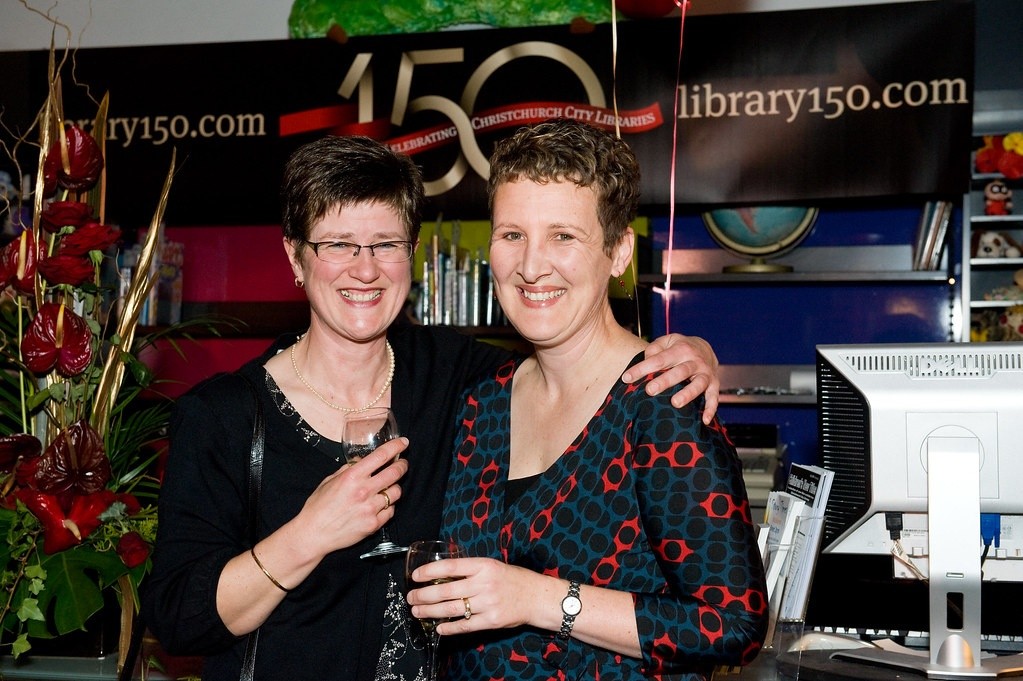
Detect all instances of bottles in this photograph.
[711,618,804,681]
[101,222,183,338]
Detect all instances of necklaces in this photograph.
[292,332,395,411]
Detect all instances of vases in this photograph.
[3,567,118,659]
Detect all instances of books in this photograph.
[420,234,509,326]
[913,200,953,271]
[755,462,835,622]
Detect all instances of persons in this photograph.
[406,116,769,681]
[142,134,719,681]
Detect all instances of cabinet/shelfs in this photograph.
[638,169,1023,405]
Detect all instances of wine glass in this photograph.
[342,407,410,558]
[405,540,463,681]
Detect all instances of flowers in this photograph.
[0,0,249,681]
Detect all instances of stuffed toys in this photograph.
[970,131,1023,343]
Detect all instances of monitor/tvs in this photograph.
[815,340,1023,681]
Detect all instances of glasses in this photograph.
[303,237,416,263]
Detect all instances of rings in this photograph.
[379,491,390,509]
[463,598,471,620]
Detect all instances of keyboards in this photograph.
[813,626,1023,652]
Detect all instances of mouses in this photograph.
[785,631,876,653]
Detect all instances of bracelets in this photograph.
[251,548,291,593]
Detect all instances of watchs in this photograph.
[557,580,582,639]
